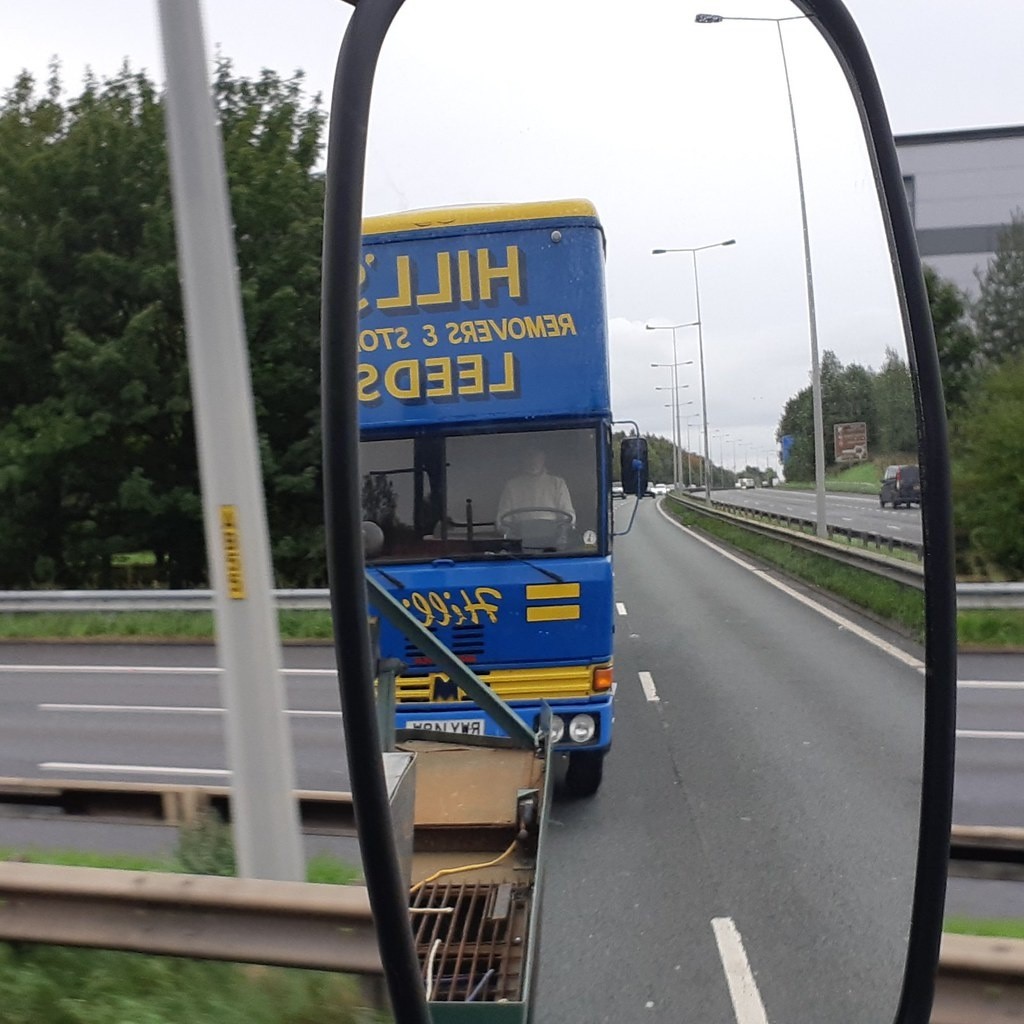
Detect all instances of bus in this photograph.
[346,195,647,805]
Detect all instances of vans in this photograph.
[878,464,923,510]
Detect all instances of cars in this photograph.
[666,484,675,493]
[655,484,668,495]
[746,479,755,488]
[609,480,626,500]
[643,480,656,499]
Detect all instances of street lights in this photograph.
[648,322,701,498]
[653,240,735,500]
[664,401,775,489]
[655,384,689,494]
[693,13,828,537]
[651,361,693,497]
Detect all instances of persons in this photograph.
[496,437,575,536]
